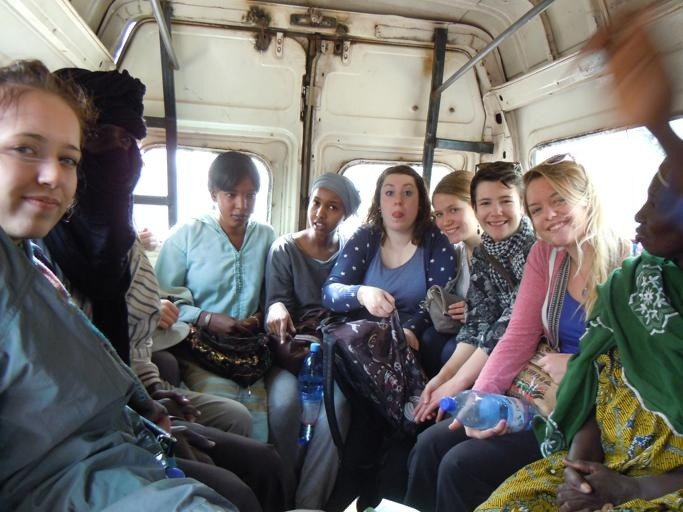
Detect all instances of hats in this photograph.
[149,313,191,353]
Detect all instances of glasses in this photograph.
[542,152,576,168]
[474,160,520,173]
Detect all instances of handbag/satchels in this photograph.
[418,284,465,334]
[271,305,330,372]
[317,309,427,458]
[507,343,573,417]
[183,330,270,389]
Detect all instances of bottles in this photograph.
[297,343,324,445]
[439,389,534,434]
[130,415,168,472]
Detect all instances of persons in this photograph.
[154,149,638,511]
[479,10,683,511]
[1,60,259,510]
